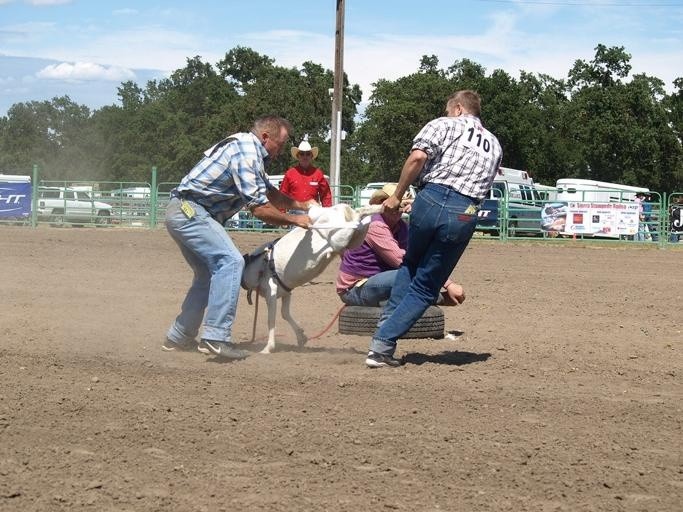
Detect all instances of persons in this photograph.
[632,192,682,243]
[364,90,504,366]
[163,115,319,360]
[277,139,333,229]
[335,182,466,309]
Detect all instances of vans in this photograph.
[0,172,30,226]
[471,166,547,240]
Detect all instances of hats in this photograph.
[383,183,414,208]
[291,141,319,161]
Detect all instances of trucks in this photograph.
[553,180,653,242]
[66,171,330,229]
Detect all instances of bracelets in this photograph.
[443,282,454,290]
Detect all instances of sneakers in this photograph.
[365,350,407,368]
[439,290,461,306]
[196,338,249,359]
[161,336,200,352]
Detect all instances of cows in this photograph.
[239,199,415,353]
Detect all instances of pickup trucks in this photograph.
[30,186,113,229]
[356,181,415,217]
